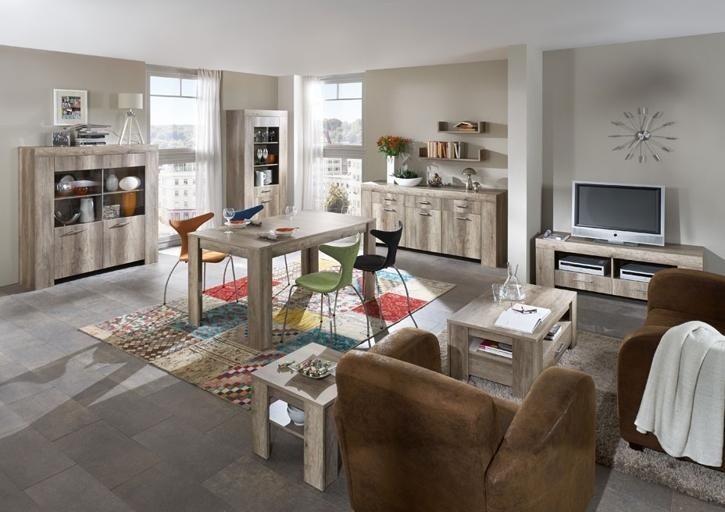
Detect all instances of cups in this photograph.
[492,283,504,305]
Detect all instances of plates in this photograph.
[224,221,251,230]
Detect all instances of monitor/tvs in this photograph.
[570,180,666,249]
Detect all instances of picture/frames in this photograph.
[53,88,88,127]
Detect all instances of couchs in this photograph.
[332,328,598,512]
[617,268,724,473]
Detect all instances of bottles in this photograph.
[500,262,525,300]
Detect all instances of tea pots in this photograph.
[255,170,266,187]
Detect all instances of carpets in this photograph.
[77,256,457,440]
[435,324,725,504]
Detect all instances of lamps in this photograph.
[117,93,145,145]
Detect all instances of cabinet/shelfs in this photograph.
[360,183,406,248]
[405,190,442,253]
[534,232,704,301]
[17,144,160,291]
[442,191,508,270]
[225,108,289,220]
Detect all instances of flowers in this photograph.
[375,135,414,158]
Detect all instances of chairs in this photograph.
[163,213,239,306]
[280,231,369,343]
[221,204,291,287]
[333,221,420,328]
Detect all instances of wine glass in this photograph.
[223,208,235,234]
[262,146,268,164]
[285,206,298,228]
[256,145,263,164]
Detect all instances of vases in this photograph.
[386,155,396,184]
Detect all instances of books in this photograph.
[477,339,513,359]
[427,141,466,159]
[544,324,562,341]
[494,303,551,334]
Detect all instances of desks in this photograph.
[186,210,377,351]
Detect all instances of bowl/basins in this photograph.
[274,227,295,238]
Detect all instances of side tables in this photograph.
[250,340,345,491]
